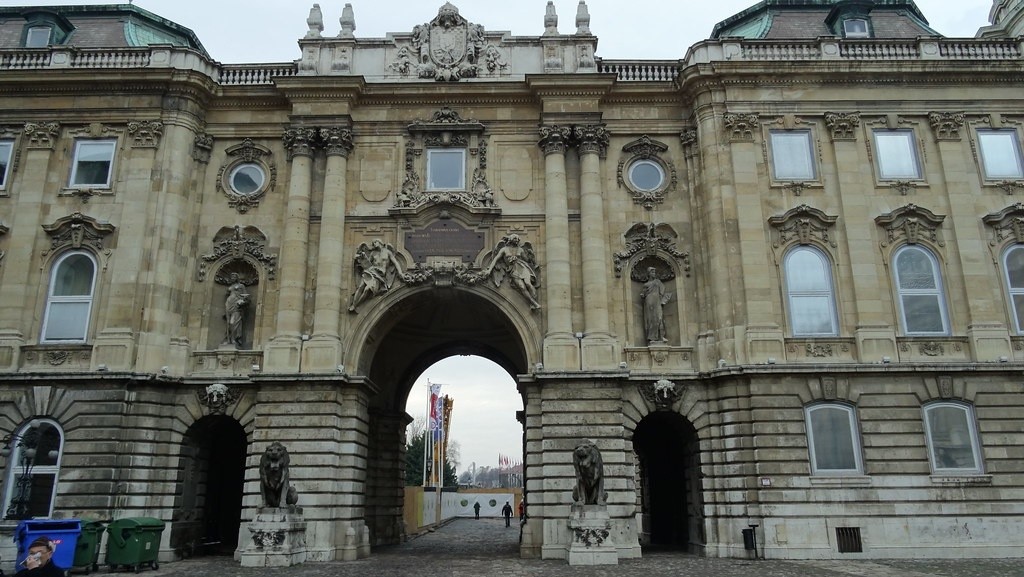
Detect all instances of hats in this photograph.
[27,536,57,553]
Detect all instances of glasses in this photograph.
[29,551,53,558]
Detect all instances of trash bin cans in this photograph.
[71,517,111,574]
[14,517,82,577]
[104,516,165,575]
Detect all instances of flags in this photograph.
[429,384,444,441]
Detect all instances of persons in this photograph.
[501,500,513,528]
[347,238,406,311]
[639,267,668,342]
[473,501,481,521]
[518,499,524,522]
[13,536,65,577]
[225,272,248,343]
[484,234,542,310]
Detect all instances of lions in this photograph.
[259,440,300,509]
[572,437,610,508]
